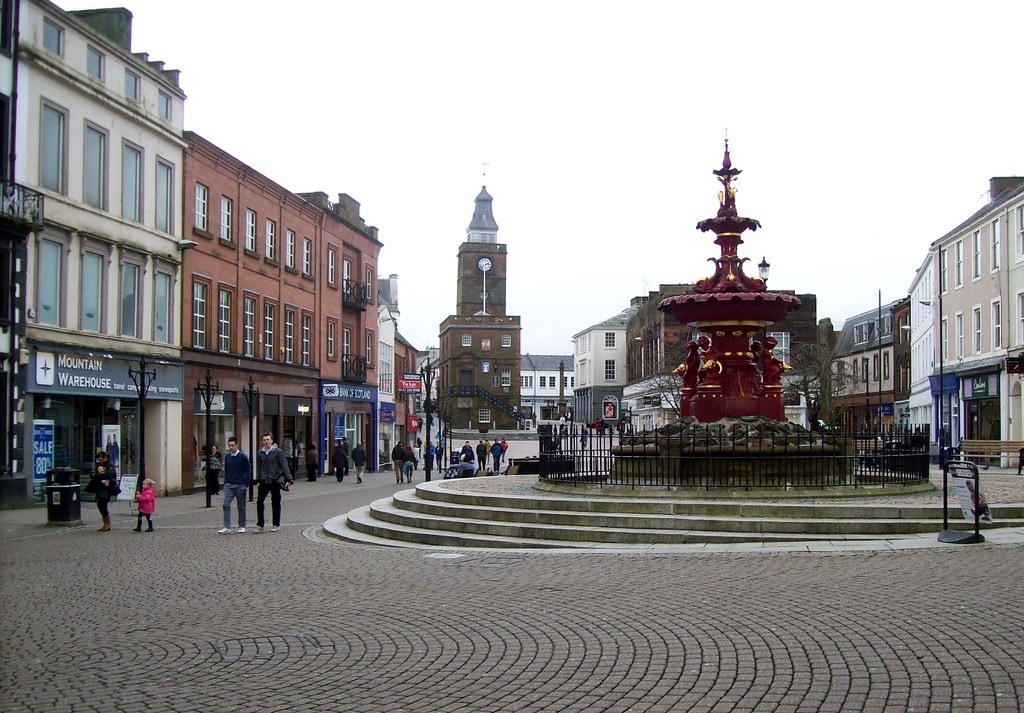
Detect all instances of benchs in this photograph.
[856,440,884,456]
[942,437,1023,474]
[500,458,576,475]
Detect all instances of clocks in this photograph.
[476,257,492,272]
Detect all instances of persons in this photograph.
[966,481,990,521]
[209,445,223,495]
[133,479,156,532]
[305,445,318,481]
[295,440,301,471]
[392,436,444,483]
[106,434,119,467]
[84,452,121,531]
[430,415,433,429]
[594,418,605,435]
[617,419,631,436]
[253,433,292,532]
[217,437,252,534]
[417,415,423,433]
[581,429,588,448]
[679,336,720,387]
[461,438,508,471]
[331,437,366,484]
[532,413,536,427]
[750,337,783,384]
[125,446,135,464]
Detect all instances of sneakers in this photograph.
[218,527,231,534]
[270,525,281,532]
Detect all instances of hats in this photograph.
[342,437,346,441]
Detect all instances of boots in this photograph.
[133,519,142,532]
[145,520,153,532]
[101,516,112,531]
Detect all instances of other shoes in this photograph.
[357,482,359,483]
[358,477,362,482]
[397,479,399,483]
[212,492,220,495]
[254,526,264,532]
[238,527,245,533]
[307,479,316,481]
[401,479,404,483]
[408,480,411,483]
[343,473,348,476]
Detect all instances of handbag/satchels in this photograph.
[276,474,289,491]
[403,464,408,475]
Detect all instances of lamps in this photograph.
[901,325,911,331]
[175,239,199,252]
[43,397,51,408]
[494,362,499,372]
[113,399,123,410]
[919,301,931,306]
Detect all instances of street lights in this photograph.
[920,299,945,469]
[193,368,220,508]
[862,351,870,437]
[421,357,435,481]
[241,373,260,501]
[127,355,157,517]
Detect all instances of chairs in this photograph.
[451,468,473,479]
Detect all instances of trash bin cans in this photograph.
[46,467,82,521]
